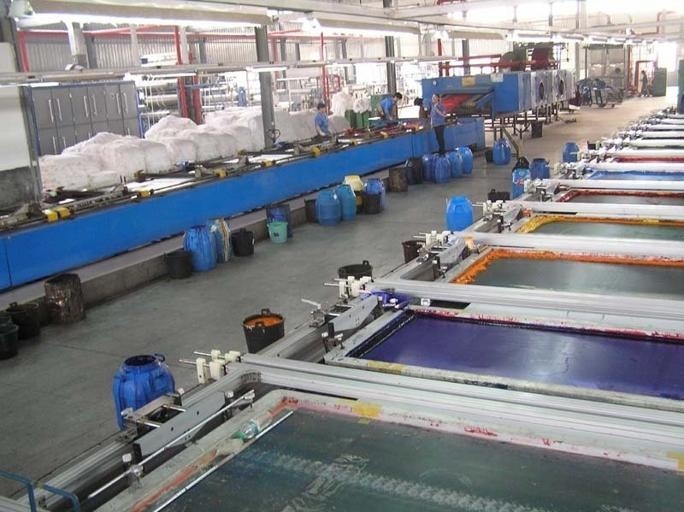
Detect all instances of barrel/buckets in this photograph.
[408,156,426,184]
[446,150,465,178]
[338,260,374,287]
[511,156,532,198]
[312,186,342,227]
[265,203,292,235]
[4,300,41,341]
[240,306,287,353]
[492,138,511,166]
[454,145,476,174]
[163,248,193,279]
[0,322,19,361]
[431,154,452,184]
[182,223,218,273]
[446,196,473,231]
[420,151,440,182]
[529,156,550,181]
[367,176,386,210]
[302,197,317,223]
[485,150,493,162]
[388,165,410,193]
[486,188,511,203]
[345,175,363,214]
[110,353,176,432]
[335,183,358,223]
[400,239,427,264]
[206,215,232,264]
[561,140,579,164]
[360,191,381,217]
[43,271,86,326]
[230,228,256,257]
[266,220,289,244]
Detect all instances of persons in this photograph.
[314,102,329,136]
[432,94,447,156]
[638,70,649,97]
[376,92,402,120]
